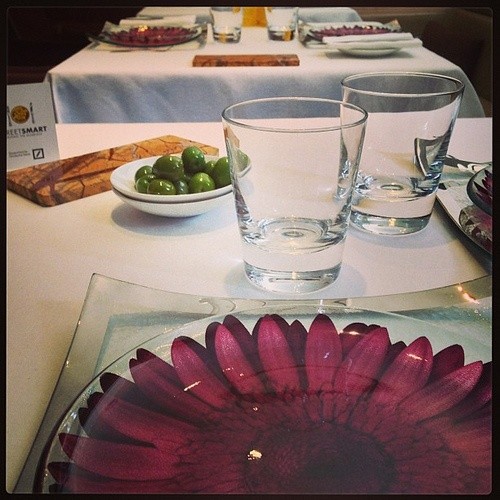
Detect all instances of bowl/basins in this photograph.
[109,154,252,219]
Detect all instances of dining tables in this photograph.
[7,100,492,494]
[137,5,363,25]
[44,19,489,124]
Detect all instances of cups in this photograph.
[338,72,466,237]
[264,7,298,41]
[220,98,370,295]
[209,7,243,44]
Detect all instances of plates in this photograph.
[39,304,492,495]
[413,138,493,256]
[337,47,401,59]
[12,271,494,496]
[302,20,394,42]
[88,26,202,48]
[466,164,493,216]
